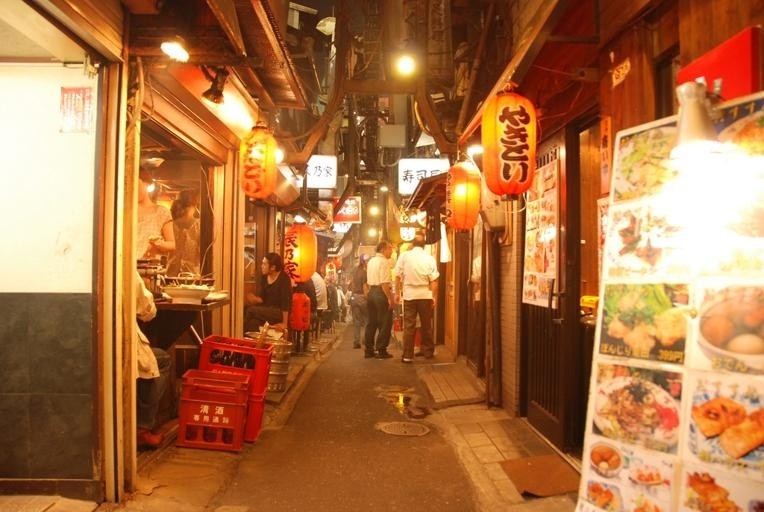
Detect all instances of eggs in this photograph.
[703,313,732,349]
[591,448,619,471]
[726,334,764,355]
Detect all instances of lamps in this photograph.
[444,83,536,232]
[399,222,417,241]
[200,64,231,105]
[239,99,275,199]
[667,78,728,163]
[159,24,193,64]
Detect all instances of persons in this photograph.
[293,234,441,362]
[471,256,485,381]
[136,270,164,447]
[243,253,290,334]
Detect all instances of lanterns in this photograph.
[240,125,278,204]
[445,156,482,232]
[480,90,539,199]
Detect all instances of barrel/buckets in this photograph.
[243,330,294,394]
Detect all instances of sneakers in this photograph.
[415,352,424,357]
[402,357,413,362]
[137,428,163,448]
[365,346,377,358]
[353,339,362,348]
[376,347,393,359]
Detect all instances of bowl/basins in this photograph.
[163,272,215,287]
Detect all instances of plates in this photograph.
[587,110,764,512]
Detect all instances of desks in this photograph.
[154,297,230,347]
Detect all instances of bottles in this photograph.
[212,349,254,368]
[137,269,155,296]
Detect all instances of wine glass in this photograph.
[159,287,210,306]
